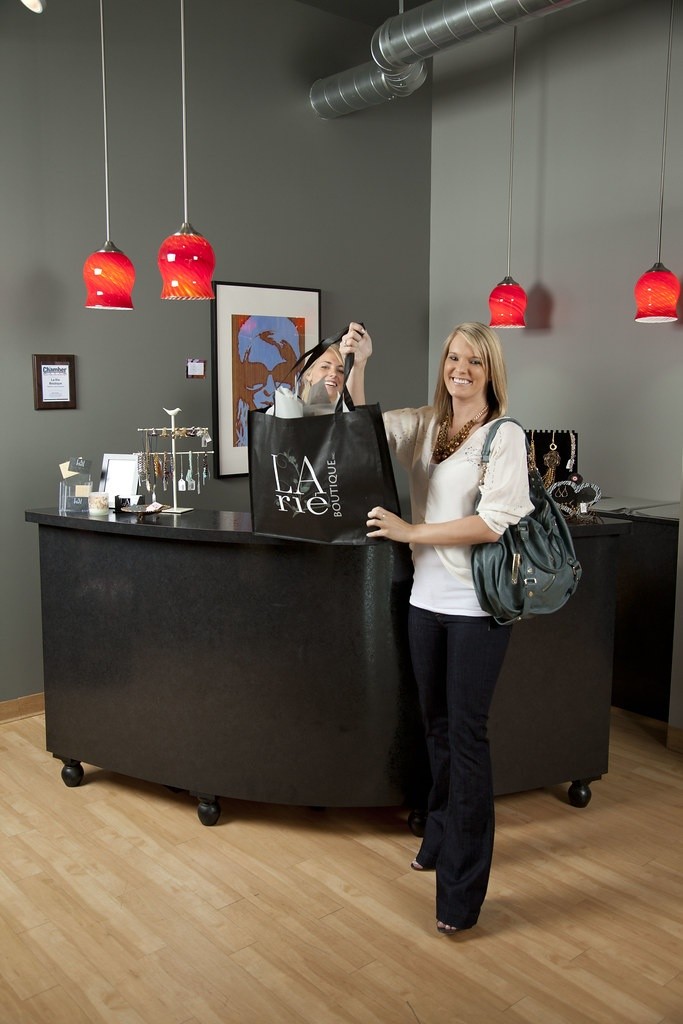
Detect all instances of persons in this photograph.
[339,321,535,933]
[299,339,344,408]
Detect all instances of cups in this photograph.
[88,492,109,516]
[75,484,93,498]
[114,494,145,513]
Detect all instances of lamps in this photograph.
[158,0,217,301]
[489,24,529,330]
[83,0,135,310]
[632,0,682,325]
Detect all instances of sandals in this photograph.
[435,919,459,935]
[411,859,433,871]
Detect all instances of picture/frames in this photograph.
[31,354,77,411]
[209,279,324,479]
[99,453,141,509]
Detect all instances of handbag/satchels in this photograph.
[470,417,583,625]
[247,320,403,545]
